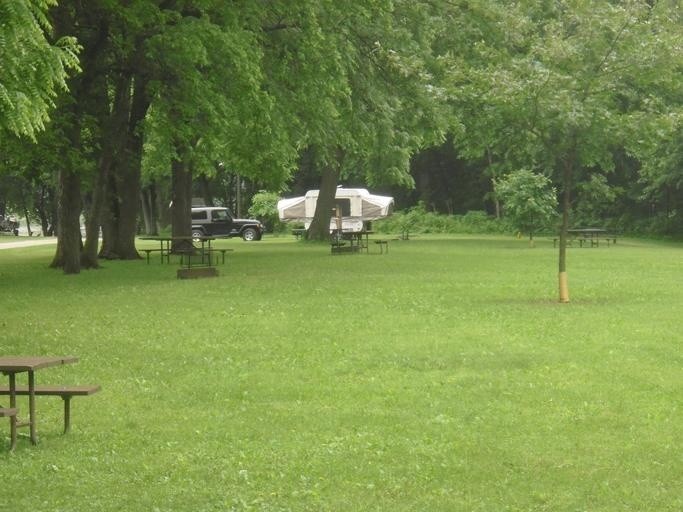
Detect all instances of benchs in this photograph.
[0,385,100,435]
[575,236,616,248]
[376,241,388,255]
[329,241,346,254]
[139,249,192,269]
[0,407,20,417]
[554,239,594,248]
[175,249,234,265]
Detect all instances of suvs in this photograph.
[190,207,264,243]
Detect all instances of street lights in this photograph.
[218,162,240,219]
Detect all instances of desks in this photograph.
[341,231,373,254]
[565,229,608,247]
[138,237,215,264]
[0,356,78,444]
